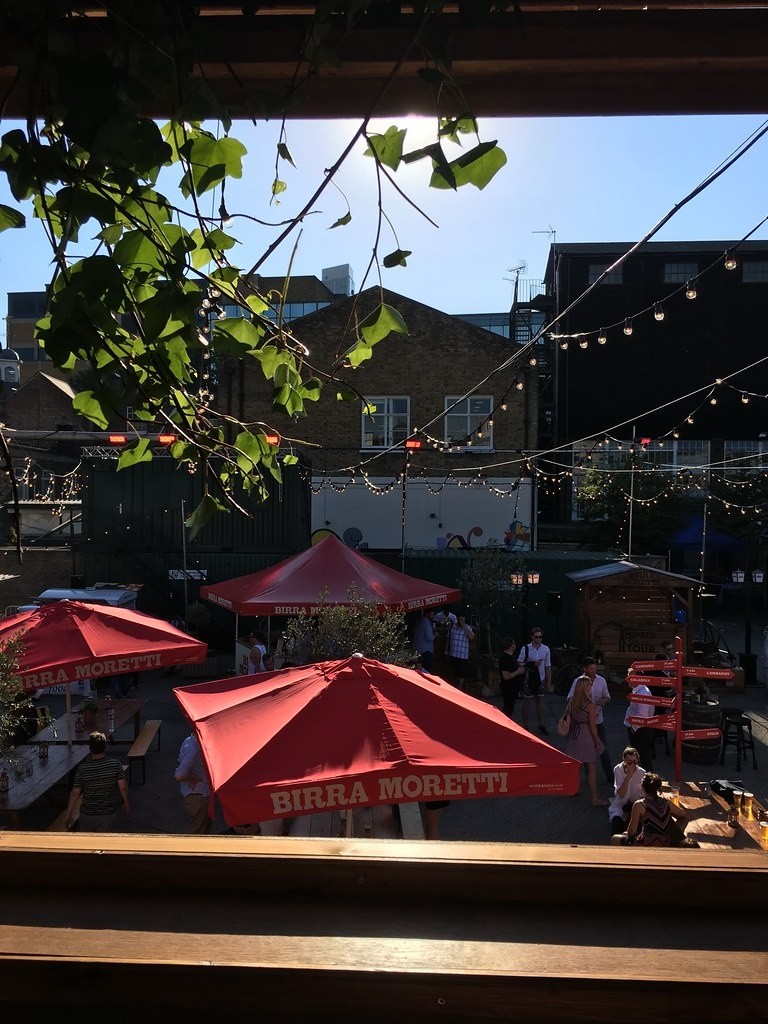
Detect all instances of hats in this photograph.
[251,631,265,641]
[89,730,108,752]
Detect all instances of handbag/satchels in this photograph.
[515,679,524,700]
[556,696,573,736]
[444,655,458,670]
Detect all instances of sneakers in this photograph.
[524,726,529,730]
[537,726,548,736]
[130,685,137,691]
[120,692,128,698]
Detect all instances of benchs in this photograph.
[45,764,129,832]
[126,720,163,787]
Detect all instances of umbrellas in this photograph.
[173,652,582,837]
[0,598,207,747]
[201,532,462,651]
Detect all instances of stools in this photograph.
[720,706,759,773]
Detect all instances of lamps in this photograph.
[265,434,280,446]
[159,434,176,444]
[109,434,126,445]
[404,439,422,448]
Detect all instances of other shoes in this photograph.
[606,776,614,782]
[608,832,612,839]
[591,799,609,807]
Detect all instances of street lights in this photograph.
[627,426,651,561]
[400,436,424,577]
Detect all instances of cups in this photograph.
[759,821,768,839]
[733,790,743,806]
[743,792,753,807]
[671,785,680,796]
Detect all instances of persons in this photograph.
[262,653,276,671]
[247,630,266,674]
[425,800,450,839]
[174,731,212,834]
[216,822,262,873]
[415,604,710,847]
[65,732,130,832]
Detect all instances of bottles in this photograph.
[75,696,94,732]
[594,744,603,755]
[108,707,115,731]
[104,692,112,720]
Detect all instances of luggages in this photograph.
[734,654,745,691]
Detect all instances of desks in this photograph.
[287,805,397,840]
[657,778,768,851]
[0,696,152,830]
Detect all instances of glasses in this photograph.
[664,647,674,652]
[249,635,255,638]
[225,668,236,673]
[623,759,639,764]
[458,618,465,621]
[533,635,543,639]
